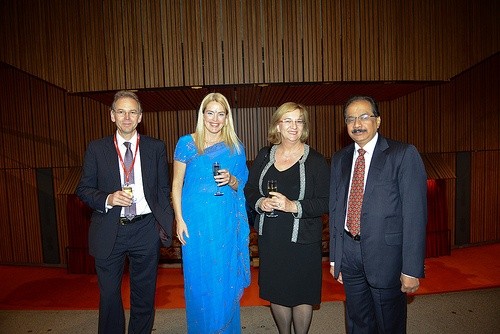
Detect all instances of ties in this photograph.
[123,142,137,221]
[346,149,366,238]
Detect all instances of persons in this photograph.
[242,102,330,334]
[171,93,251,334]
[77,92,175,334]
[329,95,428,334]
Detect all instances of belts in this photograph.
[344,229,361,241]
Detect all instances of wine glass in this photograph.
[211,161,224,196]
[122,187,136,217]
[266,178,279,218]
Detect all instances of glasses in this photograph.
[278,117,305,126]
[344,113,376,123]
[112,109,140,118]
